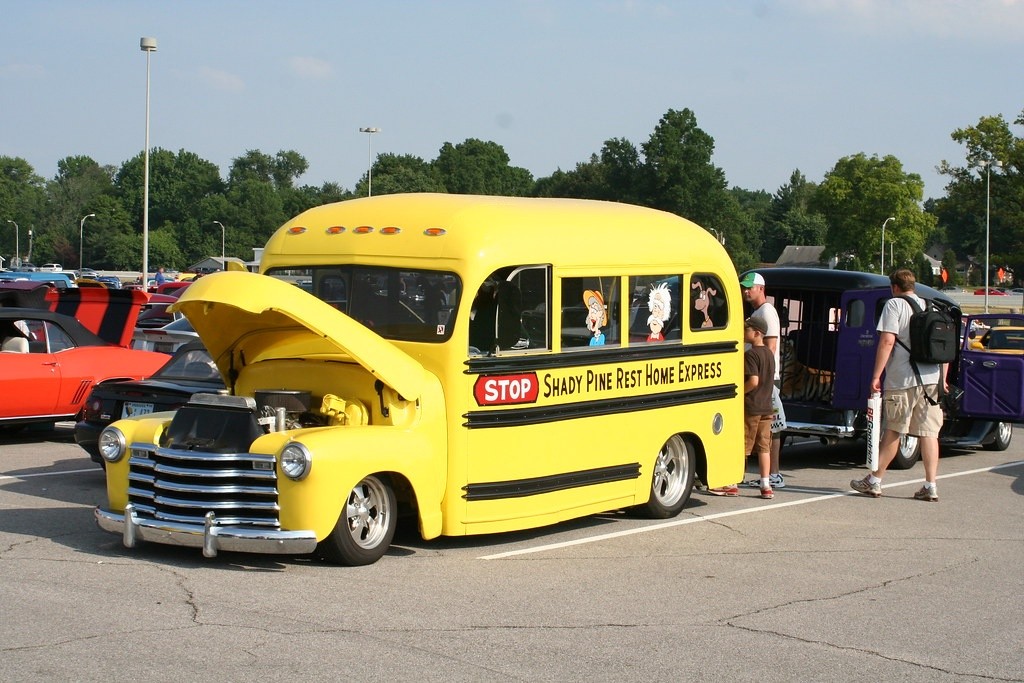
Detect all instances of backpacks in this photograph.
[894,295,955,364]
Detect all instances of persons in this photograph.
[155,267,165,286]
[708,316,774,498]
[850,270,949,501]
[740,272,788,487]
[193,268,205,282]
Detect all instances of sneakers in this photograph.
[914,485,938,500]
[749,474,785,488]
[760,485,774,498]
[708,484,738,497]
[850,478,882,498]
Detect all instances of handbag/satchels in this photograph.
[769,385,787,433]
[866,392,882,471]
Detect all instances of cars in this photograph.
[960,312,1024,355]
[0,263,228,472]
[936,285,1024,296]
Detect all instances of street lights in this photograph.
[79,214,95,278]
[139,37,158,289]
[881,217,895,277]
[7,220,18,267]
[978,154,1003,313]
[359,127,381,196]
[213,221,225,271]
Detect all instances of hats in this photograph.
[745,316,768,334]
[740,272,765,288]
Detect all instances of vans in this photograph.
[96,194,746,567]
[738,268,1024,470]
